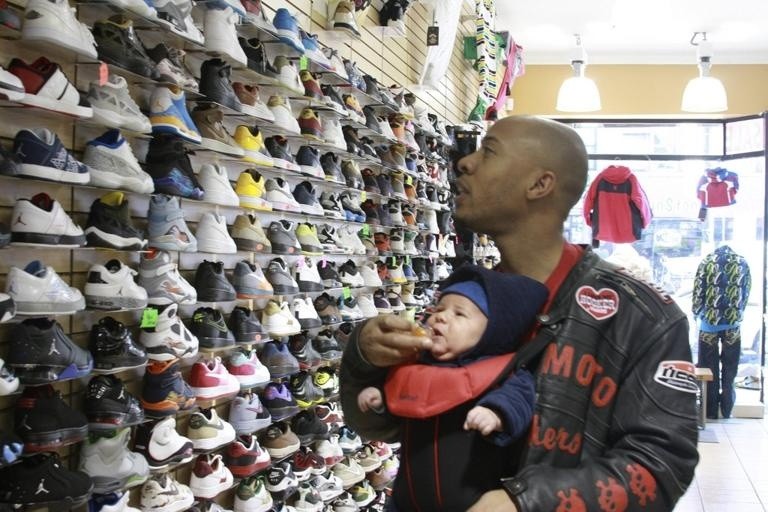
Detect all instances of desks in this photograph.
[695,366,714,425]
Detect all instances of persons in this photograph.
[694,245,752,420]
[338,115,698,512]
[357,266,550,510]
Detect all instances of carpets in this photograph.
[697,427,718,442]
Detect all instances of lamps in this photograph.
[556,33,602,113]
[680,28,729,111]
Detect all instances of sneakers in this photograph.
[233,475,274,512]
[134,248,199,308]
[193,210,238,255]
[376,126,467,228]
[272,5,306,59]
[289,295,323,330]
[9,191,89,252]
[229,392,274,437]
[341,94,368,125]
[355,292,380,319]
[197,162,241,209]
[90,11,161,82]
[299,27,332,72]
[1,1,22,43]
[189,356,242,403]
[187,453,235,501]
[317,224,355,256]
[263,256,300,296]
[193,260,238,304]
[336,427,364,454]
[359,168,381,196]
[385,286,407,312]
[241,0,280,36]
[1,357,19,399]
[311,366,341,396]
[75,425,152,496]
[136,301,200,363]
[286,445,328,482]
[296,222,326,257]
[290,255,326,293]
[233,169,273,213]
[318,150,349,187]
[87,488,144,512]
[229,212,272,255]
[337,191,368,223]
[320,117,348,152]
[364,466,392,491]
[81,126,156,195]
[140,358,197,420]
[348,479,377,507]
[335,226,368,256]
[343,57,367,93]
[143,132,205,202]
[146,80,205,145]
[207,0,247,18]
[385,255,408,285]
[232,121,274,169]
[237,33,281,82]
[272,500,296,512]
[331,455,367,489]
[358,198,381,227]
[266,218,303,256]
[292,481,325,512]
[312,433,344,466]
[333,0,362,41]
[298,69,326,105]
[403,229,500,284]
[319,82,350,119]
[374,231,394,257]
[150,41,201,94]
[0,56,94,122]
[12,383,92,447]
[8,318,95,386]
[200,502,234,512]
[357,260,383,288]
[299,109,326,144]
[289,370,326,412]
[319,189,348,222]
[0,427,25,465]
[184,405,238,456]
[380,0,412,35]
[2,290,15,326]
[84,372,147,431]
[311,328,344,361]
[271,55,306,98]
[259,421,302,461]
[373,289,393,313]
[341,124,366,159]
[366,75,438,130]
[337,158,366,192]
[226,348,272,386]
[199,57,242,113]
[232,259,275,301]
[82,190,149,252]
[6,126,90,186]
[354,445,383,474]
[85,315,149,376]
[336,294,363,323]
[289,409,331,446]
[266,94,301,137]
[1,450,94,511]
[110,0,205,50]
[313,400,345,431]
[357,226,380,256]
[129,415,195,471]
[8,259,88,319]
[315,259,342,289]
[289,180,326,218]
[87,70,153,136]
[263,459,301,502]
[21,1,103,65]
[226,431,271,479]
[404,285,435,309]
[295,145,327,182]
[260,298,302,338]
[260,340,300,378]
[357,134,383,165]
[308,469,345,504]
[260,380,299,423]
[231,79,276,124]
[200,5,249,70]
[381,455,401,477]
[229,305,271,344]
[263,132,302,176]
[337,259,365,288]
[313,291,343,327]
[285,334,322,371]
[330,491,360,512]
[190,307,236,350]
[323,45,348,80]
[83,257,148,313]
[264,177,303,216]
[139,472,197,511]
[145,192,200,255]
[373,259,395,286]
[334,322,355,349]
[369,440,393,459]
[190,102,246,160]
[389,226,407,256]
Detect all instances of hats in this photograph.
[433,280,489,317]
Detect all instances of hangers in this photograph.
[611,156,626,172]
[705,159,729,170]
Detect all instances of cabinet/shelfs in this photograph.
[0,0,503,511]
